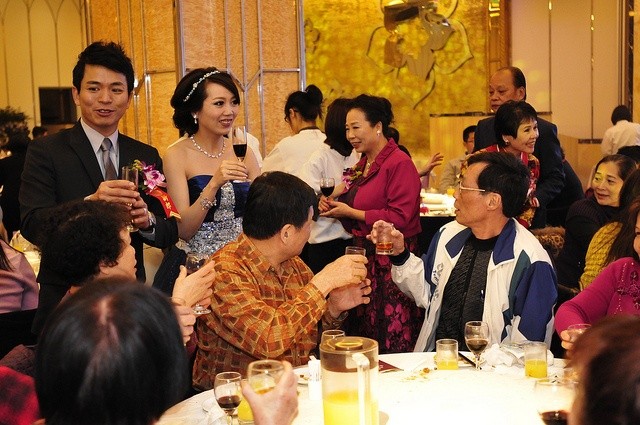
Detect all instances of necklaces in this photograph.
[367,159,374,168]
[190,134,225,159]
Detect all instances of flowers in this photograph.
[342,166,362,190]
[132,160,168,196]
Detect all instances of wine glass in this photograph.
[214,371,243,425]
[186,254,211,314]
[320,178,335,201]
[420,188,425,206]
[122,166,139,232]
[232,125,251,183]
[464,321,490,377]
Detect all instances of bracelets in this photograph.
[200,192,218,210]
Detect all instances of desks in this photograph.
[152,352,573,425]
[419,192,457,256]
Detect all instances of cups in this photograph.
[524,341,547,380]
[345,246,366,284]
[376,223,394,255]
[447,186,455,196]
[436,339,459,372]
[247,359,284,397]
[238,379,254,425]
[568,324,592,338]
[321,330,345,344]
[534,378,574,425]
[170,297,187,307]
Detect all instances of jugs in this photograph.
[319,335,380,425]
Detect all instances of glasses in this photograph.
[284,110,299,122]
[459,181,486,194]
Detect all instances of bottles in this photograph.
[12,239,23,253]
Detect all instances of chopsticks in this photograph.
[458,352,482,370]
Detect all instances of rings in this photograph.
[230,170,233,175]
[142,207,147,216]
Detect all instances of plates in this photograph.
[433,351,488,367]
[294,367,309,384]
[422,194,444,204]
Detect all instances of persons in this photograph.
[0,125,301,425]
[439,65,640,425]
[17,42,179,341]
[161,66,261,261]
[191,170,371,391]
[263,84,444,354]
[367,151,559,353]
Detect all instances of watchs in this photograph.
[140,211,156,231]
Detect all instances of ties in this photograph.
[101,138,118,181]
